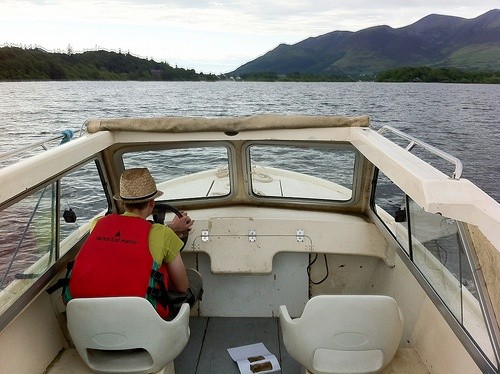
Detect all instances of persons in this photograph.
[89,166,203,320]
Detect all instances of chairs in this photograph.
[66,296,191,374]
[280,294,403,374]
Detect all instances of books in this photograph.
[227,343,280,374]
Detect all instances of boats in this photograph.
[0,116,500,372]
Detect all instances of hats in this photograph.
[112,167,164,204]
